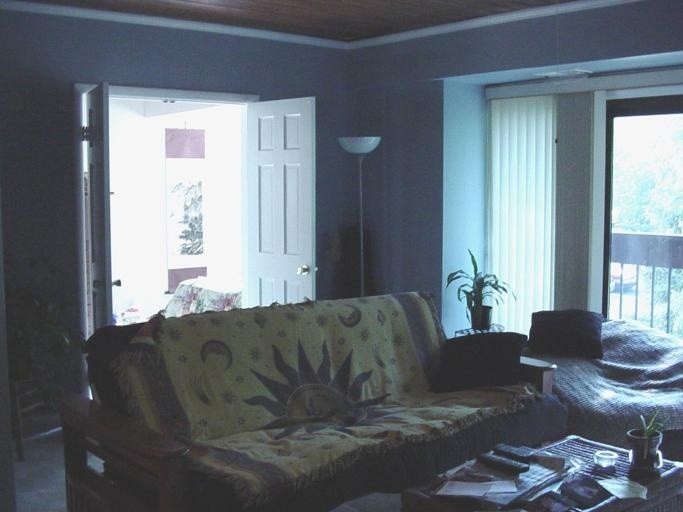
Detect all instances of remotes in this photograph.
[492,443,534,462]
[475,453,529,473]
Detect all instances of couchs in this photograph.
[63,291,570,512]
[527,312,683,461]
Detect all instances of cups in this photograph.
[594,450,619,467]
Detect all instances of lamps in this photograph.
[337,135,380,296]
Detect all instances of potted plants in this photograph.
[623,410,670,470]
[445,248,514,328]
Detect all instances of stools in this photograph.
[9,383,63,462]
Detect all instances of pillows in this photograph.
[528,310,605,359]
[428,332,527,392]
